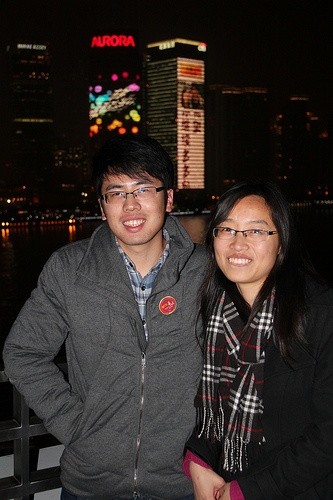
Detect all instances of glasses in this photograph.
[211,225,279,241]
[98,185,166,203]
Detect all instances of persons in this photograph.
[184,181,333,499]
[4,135,216,500]
